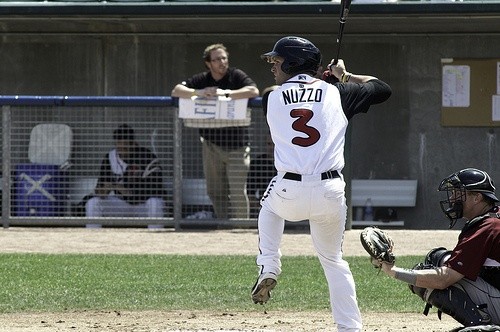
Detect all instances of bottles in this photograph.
[364,198,374,221]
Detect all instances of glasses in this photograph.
[209,56,230,63]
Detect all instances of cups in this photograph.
[356,207,363,221]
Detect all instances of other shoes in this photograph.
[457,321,500,332]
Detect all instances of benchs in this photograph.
[73,175,419,226]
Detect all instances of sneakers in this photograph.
[251,272,277,305]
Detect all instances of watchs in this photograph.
[224,89,231,98]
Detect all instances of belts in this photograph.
[283,170,340,181]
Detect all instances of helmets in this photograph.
[454,168,499,201]
[263,36,322,76]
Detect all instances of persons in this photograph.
[250,36,393,332]
[171,43,260,229]
[359,168,500,332]
[85,122,168,228]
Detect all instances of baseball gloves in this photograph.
[333,0,352,65]
[360,227,397,268]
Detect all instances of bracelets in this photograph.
[340,70,353,84]
